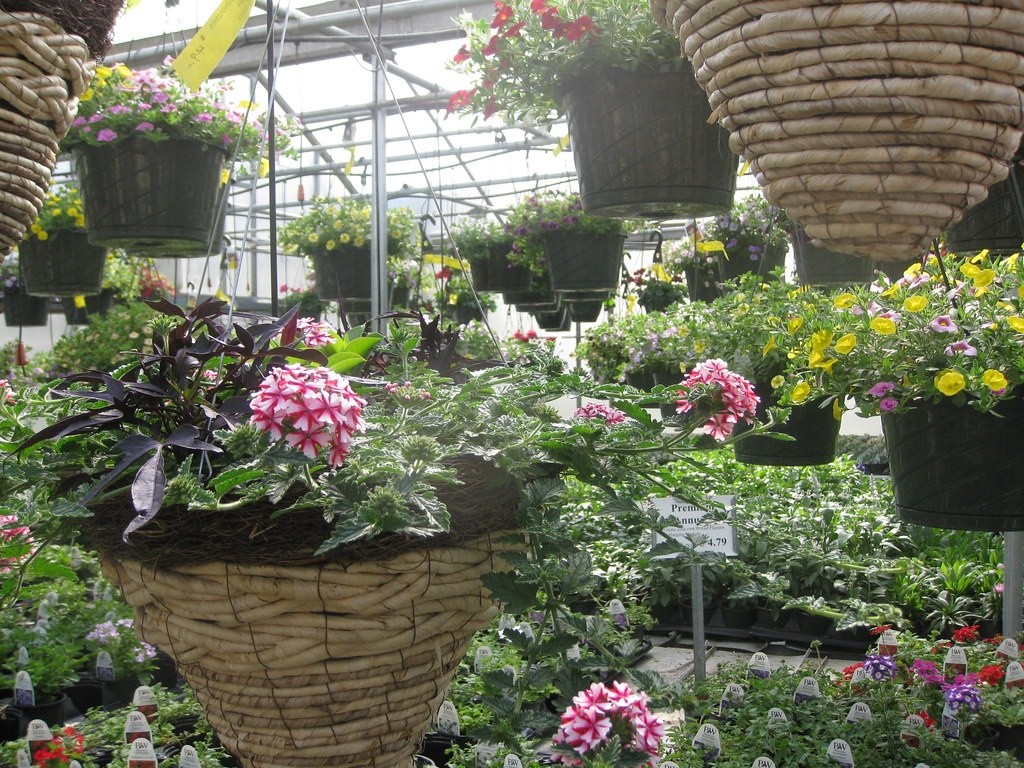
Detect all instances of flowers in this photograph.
[10,297,544,553]
[764,241,1024,417]
[504,194,587,276]
[454,216,514,263]
[384,253,434,289]
[0,251,21,293]
[23,180,88,242]
[841,623,1024,747]
[282,196,421,259]
[632,263,689,305]
[61,61,300,173]
[551,682,665,768]
[579,272,831,388]
[715,196,789,261]
[444,0,685,126]
[661,223,722,274]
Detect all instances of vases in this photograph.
[99,530,537,768]
[881,384,1024,531]
[312,250,339,300]
[567,301,603,322]
[626,370,660,408]
[65,132,231,252]
[388,282,410,310]
[561,290,609,302]
[730,242,785,283]
[733,386,845,466]
[60,288,112,326]
[646,299,672,314]
[337,244,371,301]
[686,263,733,302]
[967,721,1024,757]
[18,226,106,297]
[472,259,572,333]
[133,161,230,258]
[3,287,49,326]
[556,58,738,221]
[652,370,696,426]
[541,228,629,293]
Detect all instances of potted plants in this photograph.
[649,560,884,645]
